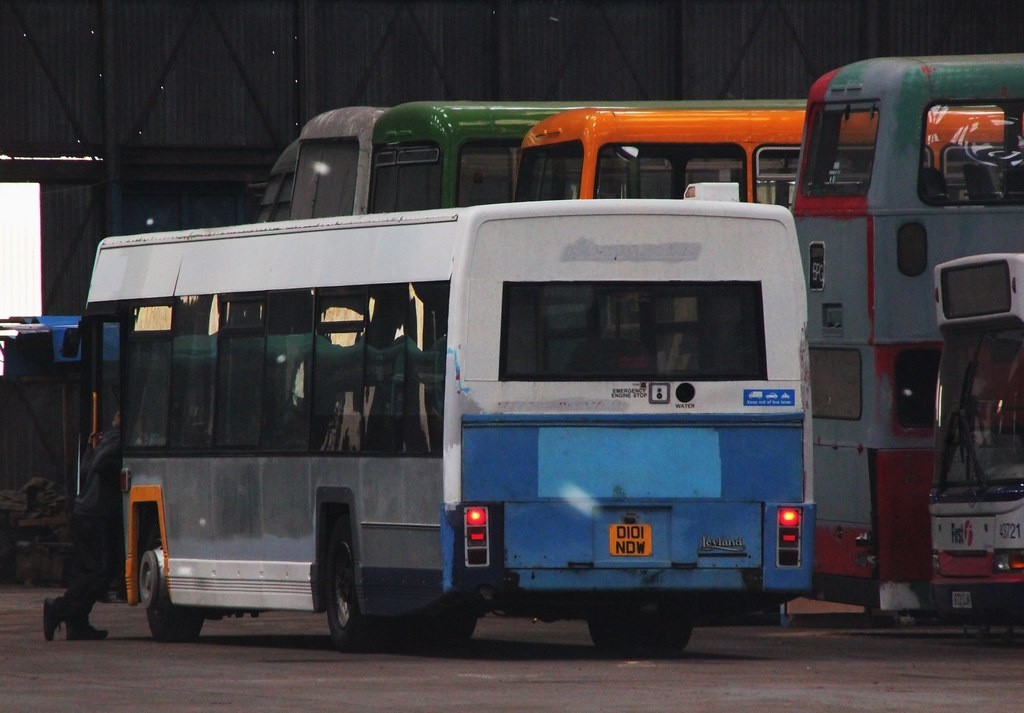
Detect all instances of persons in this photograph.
[43,410,120,641]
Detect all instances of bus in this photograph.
[58,177,818,661]
[502,105,1012,205]
[928,253,1023,638]
[287,96,388,217]
[365,96,807,218]
[788,49,1024,606]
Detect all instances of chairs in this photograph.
[963,162,997,200]
[921,169,947,202]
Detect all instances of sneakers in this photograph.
[65,614,108,641]
[43,597,64,640]
